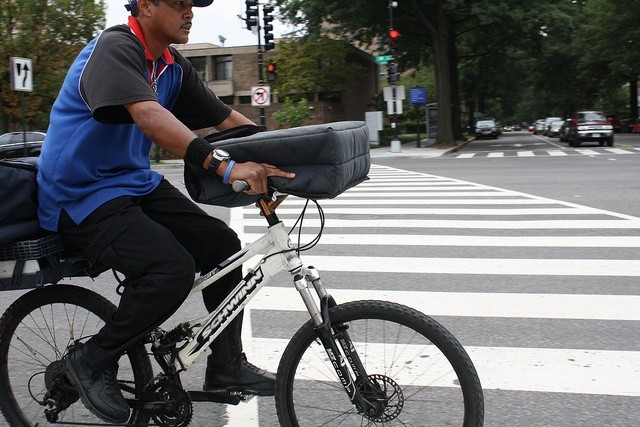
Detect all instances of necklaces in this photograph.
[151,60,158,93]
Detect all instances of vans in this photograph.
[484,116,501,135]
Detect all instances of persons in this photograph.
[35,1,294,423]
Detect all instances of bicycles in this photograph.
[1,177,486,426]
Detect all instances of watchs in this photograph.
[206,145,231,174]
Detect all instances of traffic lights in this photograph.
[387,28,400,56]
[245,0,258,31]
[264,4,274,51]
[266,61,275,83]
[389,63,400,82]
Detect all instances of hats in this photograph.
[124,0,214,11]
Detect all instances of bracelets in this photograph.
[223,159,236,184]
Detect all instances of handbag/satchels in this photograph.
[1,157,42,246]
[184,121,370,207]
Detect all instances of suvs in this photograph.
[606,113,624,134]
[544,117,562,136]
[569,110,615,147]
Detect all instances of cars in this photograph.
[0,132,45,156]
[549,120,565,138]
[504,125,512,132]
[514,124,522,131]
[474,120,498,139]
[560,118,573,142]
[528,122,535,131]
[536,119,544,134]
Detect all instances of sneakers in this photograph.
[203,353,277,396]
[64,342,130,423]
